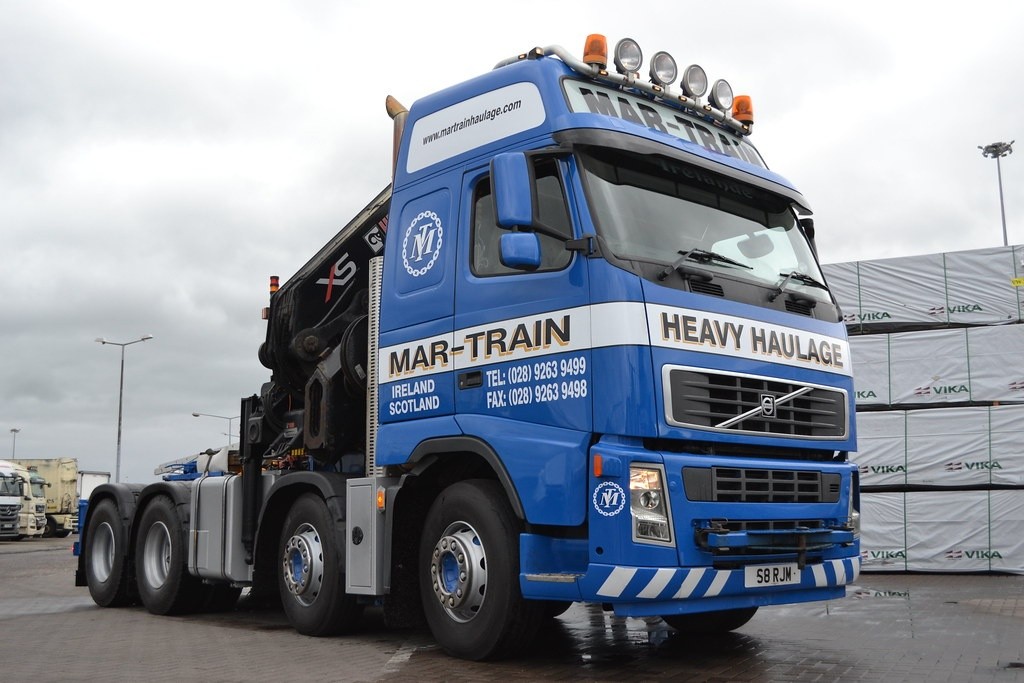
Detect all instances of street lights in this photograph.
[978,139,1015,247]
[94,333,154,484]
[192,413,240,446]
[10,427,21,459]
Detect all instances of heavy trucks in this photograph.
[0,457,77,542]
[72,32,864,662]
